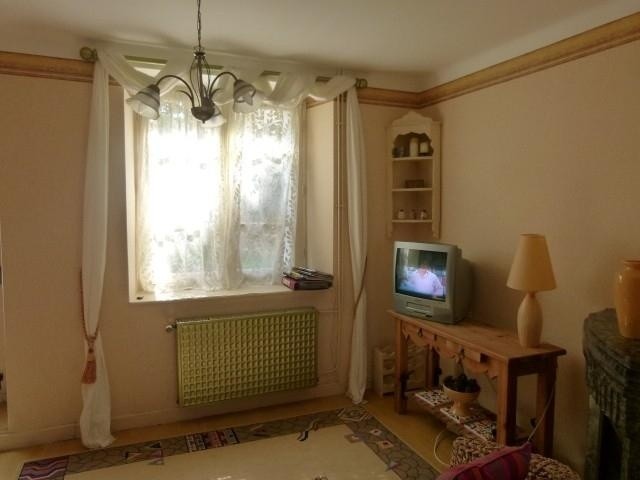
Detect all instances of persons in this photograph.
[406,259,442,297]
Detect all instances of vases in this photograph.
[614,259,640,339]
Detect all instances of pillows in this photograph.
[436,441,531,479]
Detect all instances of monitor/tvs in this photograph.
[391,240,475,324]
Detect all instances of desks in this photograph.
[582,308,639,480]
[384,309,567,459]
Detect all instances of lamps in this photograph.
[126,0,265,127]
[506,234,556,348]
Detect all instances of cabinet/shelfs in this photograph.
[385,109,442,239]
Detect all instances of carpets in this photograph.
[12,404,442,480]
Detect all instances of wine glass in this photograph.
[443,375,481,417]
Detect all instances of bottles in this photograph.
[409,137,419,157]
[399,209,428,220]
